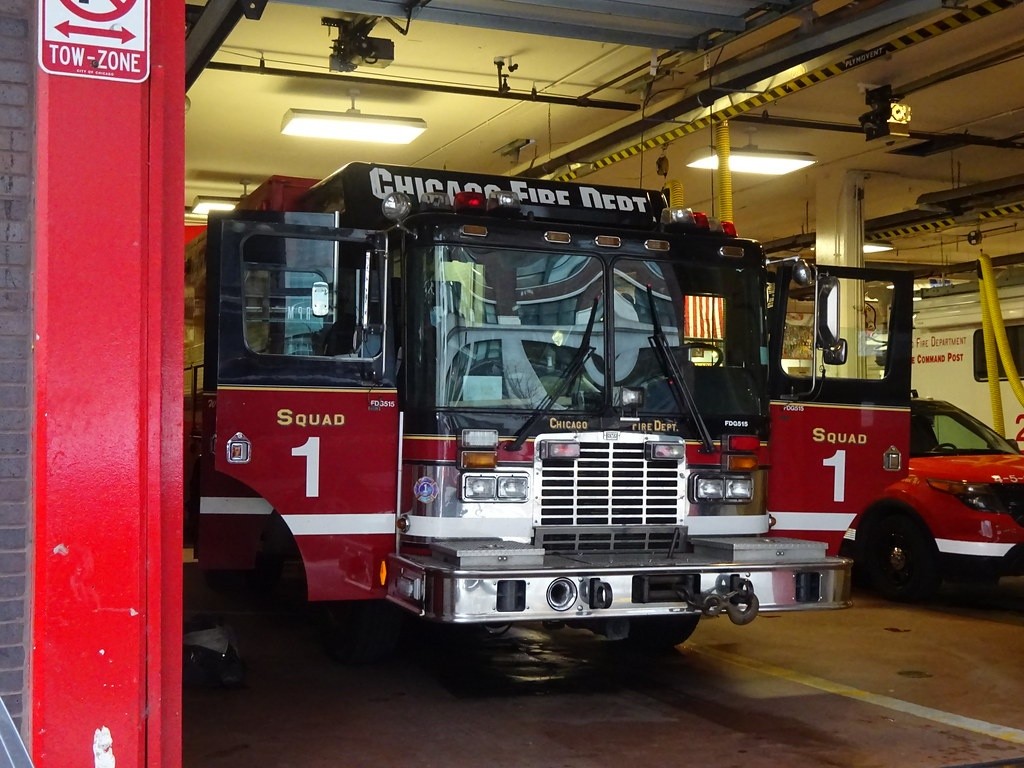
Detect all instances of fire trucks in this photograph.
[183,159,920,700]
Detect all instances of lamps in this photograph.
[278,83,428,145]
[191,178,249,219]
[811,184,896,256]
[686,125,819,178]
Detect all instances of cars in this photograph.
[841,382,1024,594]
